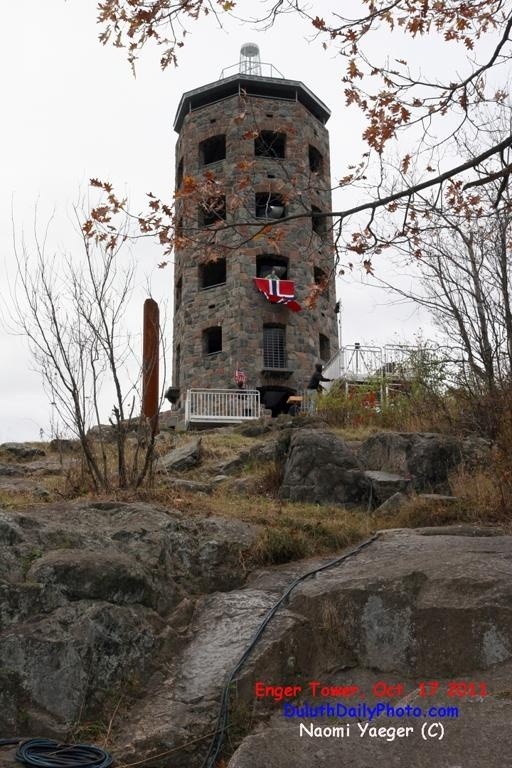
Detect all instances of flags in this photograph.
[254,279,303,312]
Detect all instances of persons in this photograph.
[307,363,335,415]
[264,270,280,280]
